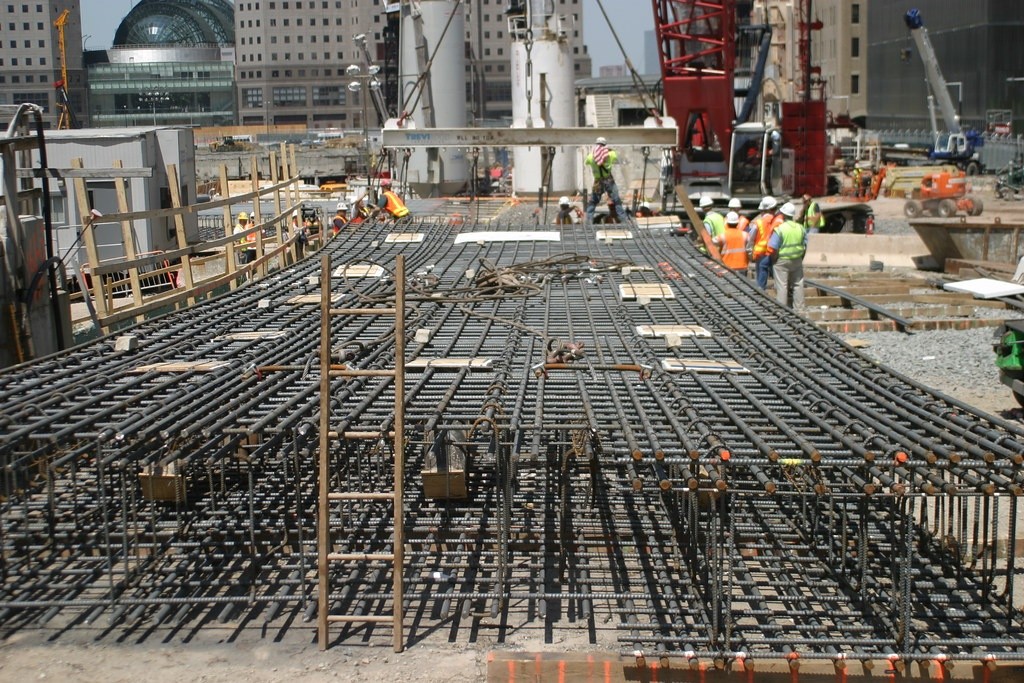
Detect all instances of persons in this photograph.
[853,162,862,198]
[699,193,824,311]
[291,210,306,257]
[334,203,348,237]
[233,212,264,276]
[557,196,580,224]
[585,137,628,225]
[378,180,409,224]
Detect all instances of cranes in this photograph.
[652,0,737,207]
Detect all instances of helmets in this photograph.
[728,198,741,207]
[699,196,713,206]
[337,203,348,211]
[762,196,776,210]
[802,194,811,202]
[758,202,763,210]
[727,212,739,224]
[237,212,248,219]
[250,212,254,217]
[292,210,298,218]
[559,196,569,204]
[780,203,794,216]
[596,137,607,145]
[380,180,392,187]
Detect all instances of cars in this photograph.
[299,140,322,146]
[284,138,302,144]
[319,184,347,196]
[260,184,322,199]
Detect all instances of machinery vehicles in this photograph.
[53,79,80,129]
[727,0,885,234]
[901,9,984,176]
[905,172,983,219]
[932,256,1024,410]
[300,203,324,234]
[351,34,391,129]
[209,134,245,152]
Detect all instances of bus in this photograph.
[233,135,252,142]
[317,133,343,142]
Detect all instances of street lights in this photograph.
[264,100,270,140]
[346,65,381,200]
[139,87,170,126]
